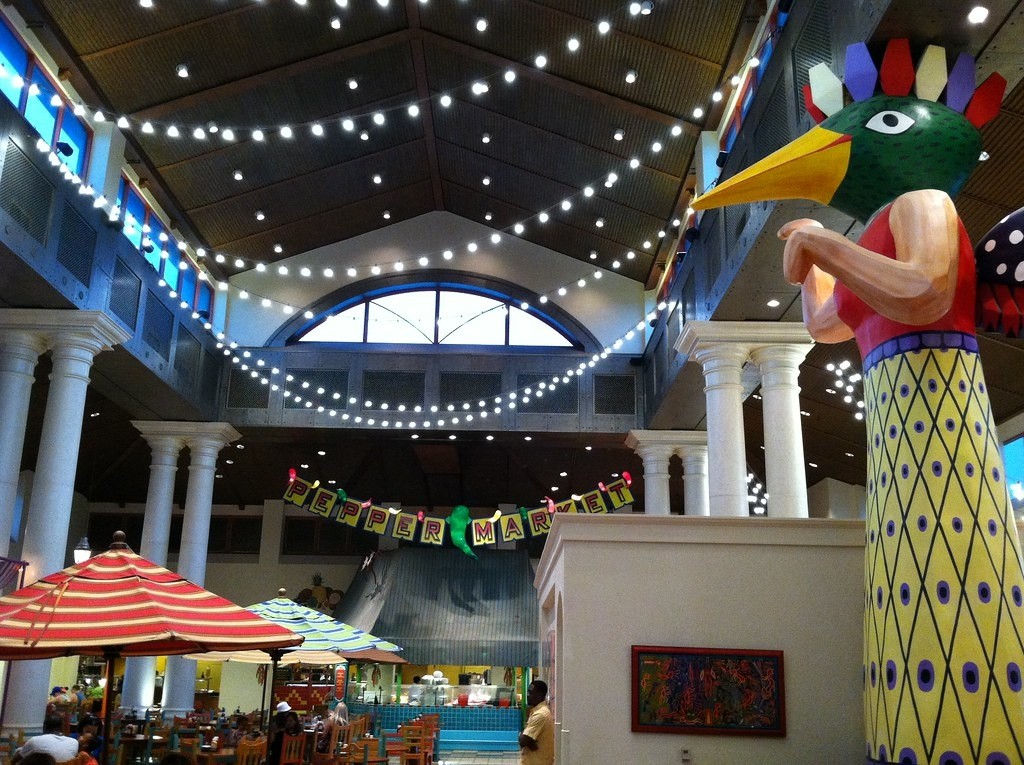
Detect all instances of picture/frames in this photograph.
[629,645,789,738]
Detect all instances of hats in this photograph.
[62,687,67,692]
[50,686,62,695]
[277,702,292,713]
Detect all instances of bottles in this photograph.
[211,738,218,749]
[8,736,17,758]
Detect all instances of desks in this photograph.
[408,727,440,737]
[120,717,157,731]
[351,755,387,764]
[178,719,252,727]
[381,745,409,764]
[155,724,211,739]
[302,730,326,762]
[406,734,435,762]
[170,746,250,764]
[109,732,166,764]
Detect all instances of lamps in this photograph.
[139,241,154,255]
[715,150,729,170]
[651,319,657,327]
[675,250,687,263]
[55,140,73,157]
[196,310,210,320]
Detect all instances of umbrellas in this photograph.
[183,651,348,732]
[332,649,410,704]
[0,531,305,765]
[245,588,404,765]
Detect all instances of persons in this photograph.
[12,685,104,765]
[317,702,348,754]
[409,676,423,703]
[268,701,298,765]
[518,680,554,765]
[229,716,249,745]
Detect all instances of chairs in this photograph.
[1,699,442,765]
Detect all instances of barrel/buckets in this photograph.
[458,694,469,708]
[499,697,510,709]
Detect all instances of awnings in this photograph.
[329,549,540,668]
